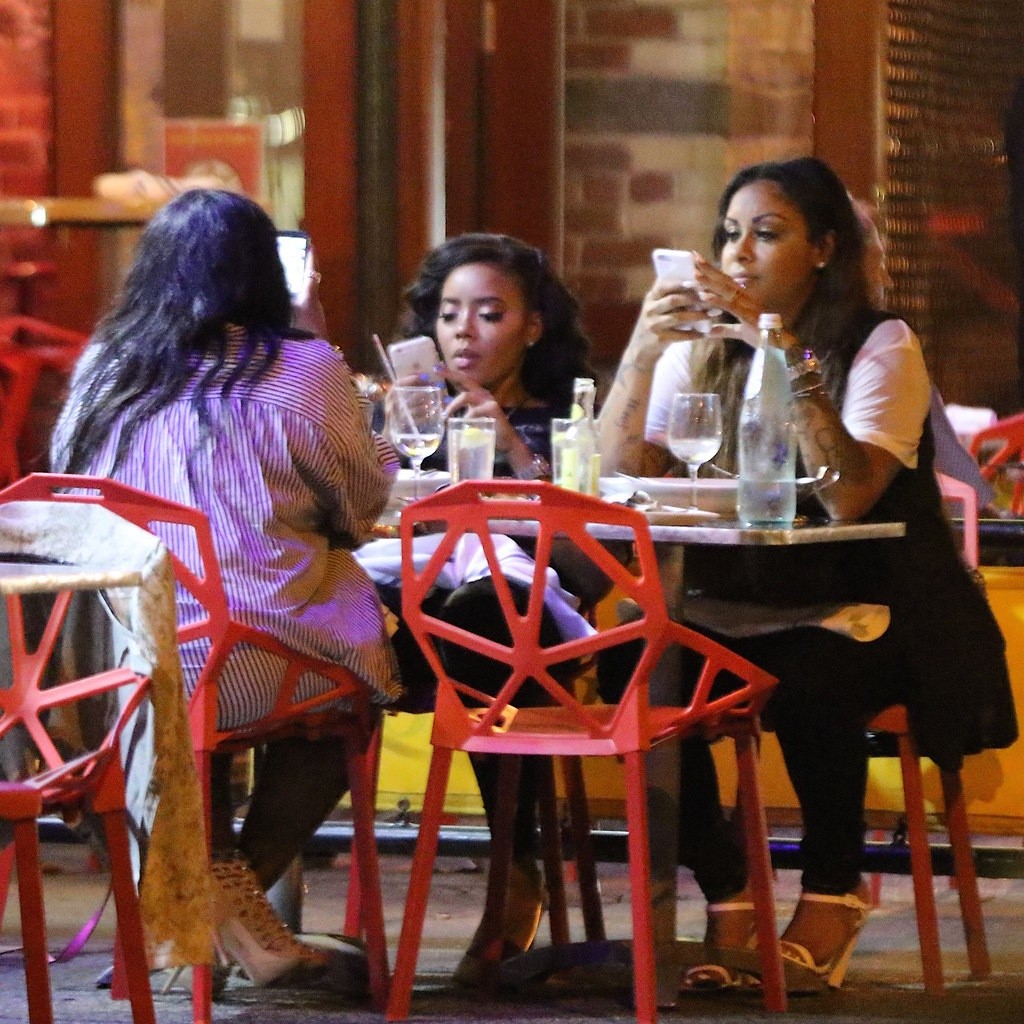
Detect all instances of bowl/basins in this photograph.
[600,477,734,510]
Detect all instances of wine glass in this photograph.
[388,387,445,499]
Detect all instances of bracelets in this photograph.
[783,345,834,404]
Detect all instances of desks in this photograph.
[0,561,143,594]
[380,519,911,1015]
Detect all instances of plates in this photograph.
[393,468,450,486]
[644,505,724,524]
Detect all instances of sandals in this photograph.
[446,868,545,999]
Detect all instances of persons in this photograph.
[594,156,1019,998]
[48,191,397,995]
[372,231,599,988]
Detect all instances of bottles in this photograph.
[563,378,598,497]
[737,312,796,522]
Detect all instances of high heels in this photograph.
[202,858,326,987]
[680,902,757,995]
[740,879,873,996]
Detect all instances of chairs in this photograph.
[0,473,386,1024]
[760,469,993,999]
[971,414,1024,517]
[386,478,788,1024]
[0,498,176,1024]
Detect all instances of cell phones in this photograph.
[277,231,312,307]
[389,336,454,429]
[650,248,715,333]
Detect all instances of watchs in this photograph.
[511,454,554,486]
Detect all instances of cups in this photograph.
[552,418,598,494]
[667,394,723,513]
[447,418,495,485]
